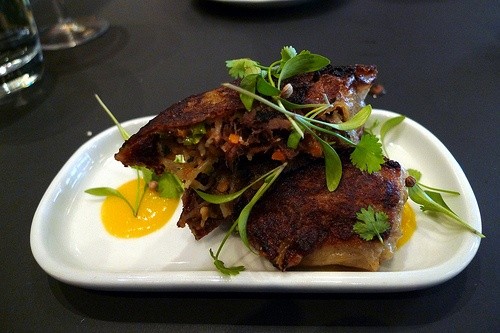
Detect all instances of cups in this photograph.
[0,0,44,98]
[30,0,110,51]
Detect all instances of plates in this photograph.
[30,109,482,293]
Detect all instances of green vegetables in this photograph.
[85,45,486,276]
